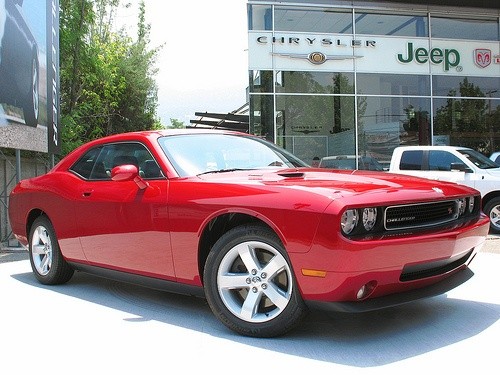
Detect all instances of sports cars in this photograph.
[7,127,491,337]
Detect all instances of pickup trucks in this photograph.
[317,154,385,174]
[349,145,500,235]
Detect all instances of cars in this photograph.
[0,0,40,126]
